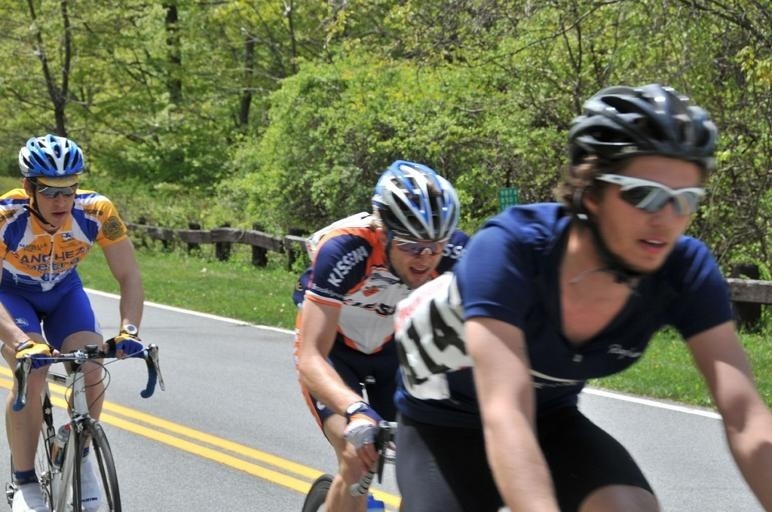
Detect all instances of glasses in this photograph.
[26,177,79,199]
[390,236,445,258]
[592,170,707,217]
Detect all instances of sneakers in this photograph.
[10,480,50,512]
[72,459,102,512]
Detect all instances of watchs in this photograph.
[341,402,377,421]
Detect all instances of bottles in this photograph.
[51,424,71,470]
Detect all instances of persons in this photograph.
[387,86,772,512]
[0,133,143,512]
[291,161,474,512]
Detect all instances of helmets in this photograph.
[19,135,85,178]
[372,158,460,244]
[565,82,719,173]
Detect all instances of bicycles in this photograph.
[5,340,165,512]
[300,416,403,512]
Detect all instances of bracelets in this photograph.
[117,324,138,336]
